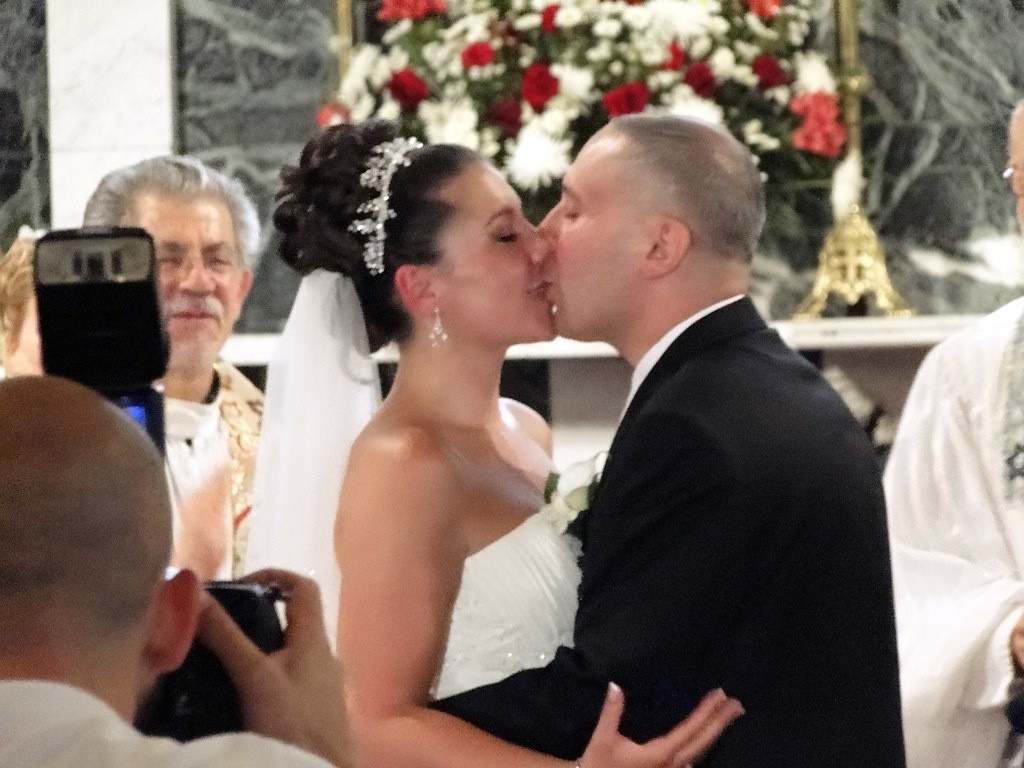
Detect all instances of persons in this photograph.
[273,114,908,768]
[882,97,1024,768]
[0,156,357,768]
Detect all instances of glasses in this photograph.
[1002,161,1024,197]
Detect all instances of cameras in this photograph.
[34,224,287,745]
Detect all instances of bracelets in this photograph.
[575,759,580,768]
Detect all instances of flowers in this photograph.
[552,449,610,533]
[315,0,854,255]
